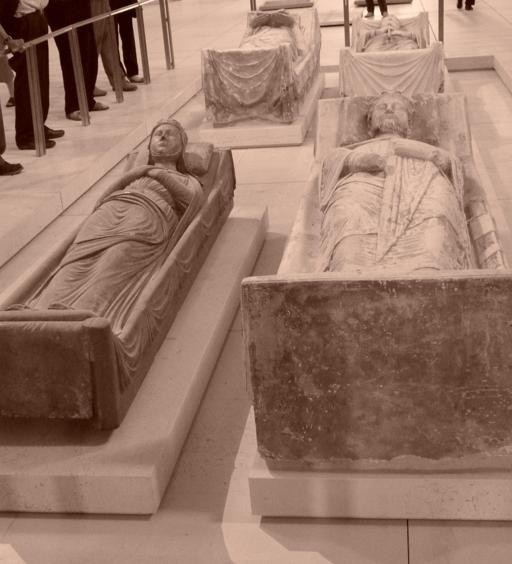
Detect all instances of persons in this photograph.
[356,13,420,53]
[316,89,473,278]
[238,7,298,66]
[1,0,148,178]
[363,0,389,16]
[455,0,475,10]
[4,115,204,323]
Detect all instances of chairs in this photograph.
[365,12,374,17]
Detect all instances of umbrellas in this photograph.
[0,75,144,176]
